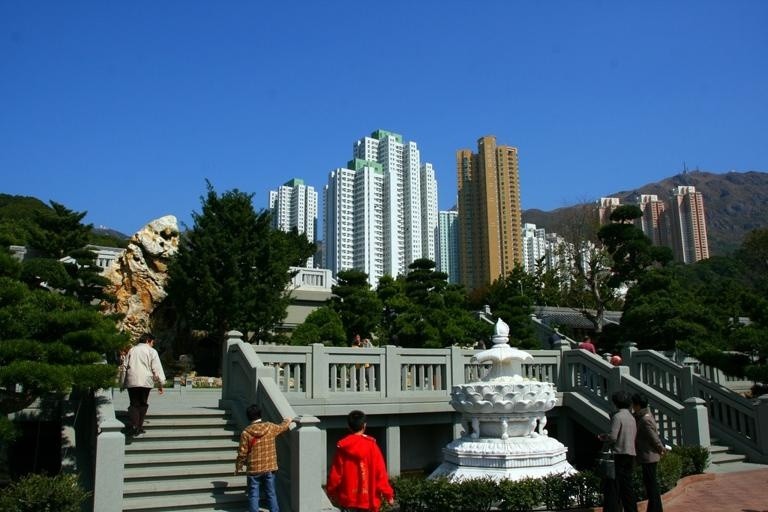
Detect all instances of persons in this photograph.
[327,409,395,511]
[577,336,596,388]
[628,392,667,512]
[118,329,167,438]
[609,352,623,366]
[233,403,294,512]
[596,389,642,511]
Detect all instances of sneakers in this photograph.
[128,422,145,437]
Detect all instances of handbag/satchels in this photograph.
[591,435,618,480]
